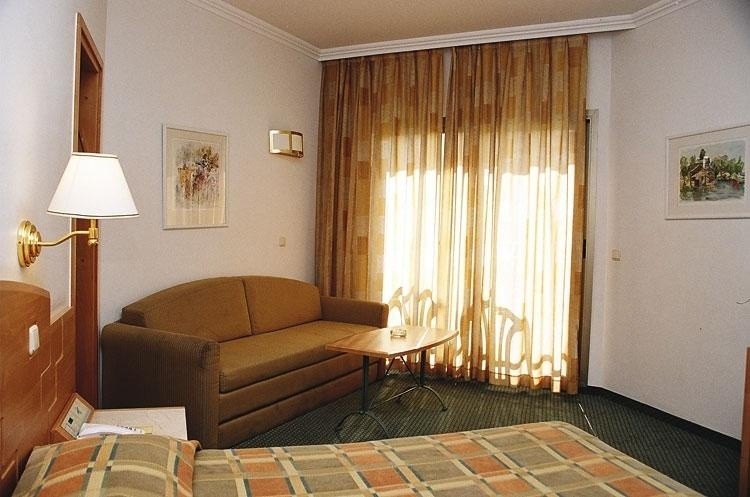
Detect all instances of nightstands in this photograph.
[52,393,188,440]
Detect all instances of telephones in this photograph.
[78,423,145,437]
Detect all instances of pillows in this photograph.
[7,434,201,497]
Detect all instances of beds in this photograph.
[1,281,711,496]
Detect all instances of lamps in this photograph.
[269,130,304,158]
[17,151,141,269]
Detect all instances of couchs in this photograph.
[100,275,389,448]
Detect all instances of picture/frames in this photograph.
[162,123,228,231]
[664,123,750,219]
